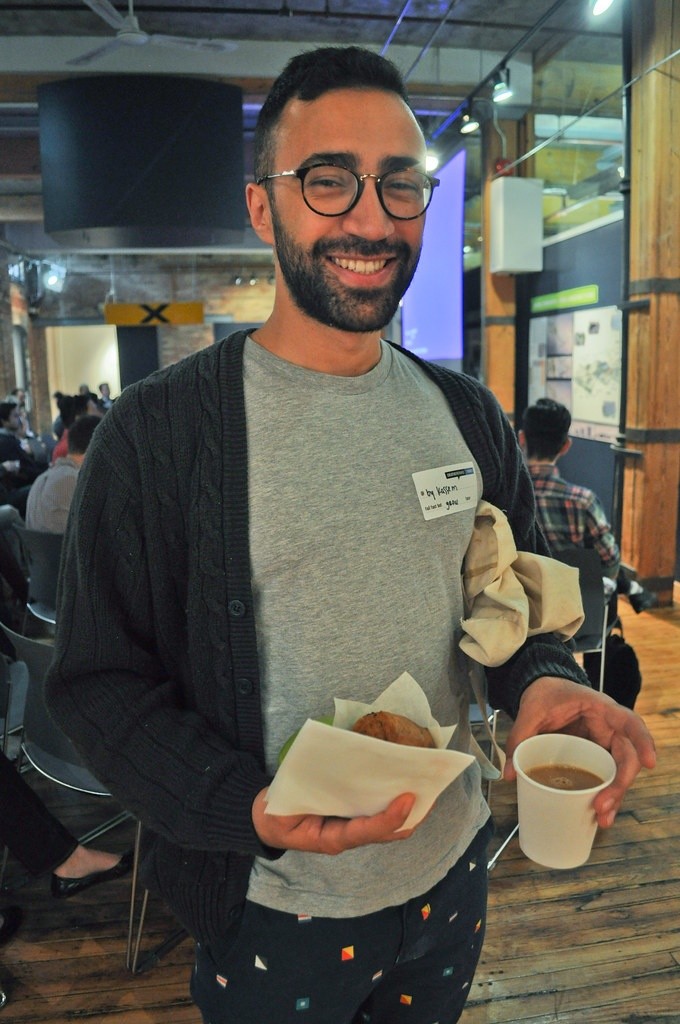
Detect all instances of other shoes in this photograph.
[610,616,624,639]
[628,585,659,614]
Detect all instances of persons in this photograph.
[47,47,657,1024]
[1,385,657,1023]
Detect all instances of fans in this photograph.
[66,0,238,70]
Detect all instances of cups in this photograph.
[513,732,617,868]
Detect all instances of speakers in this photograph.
[489,177,544,274]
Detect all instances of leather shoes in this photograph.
[51,847,134,898]
[0,904,23,945]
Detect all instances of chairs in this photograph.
[27,436,49,466]
[470,703,519,876]
[41,429,57,462]
[0,505,36,636]
[2,520,64,638]
[0,652,30,770]
[551,546,623,693]
[1,623,185,971]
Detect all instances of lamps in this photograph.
[460,108,479,135]
[234,266,275,286]
[38,259,67,293]
[7,258,42,302]
[492,62,514,103]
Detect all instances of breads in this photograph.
[351,711,435,748]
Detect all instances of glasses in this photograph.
[254,162,441,220]
